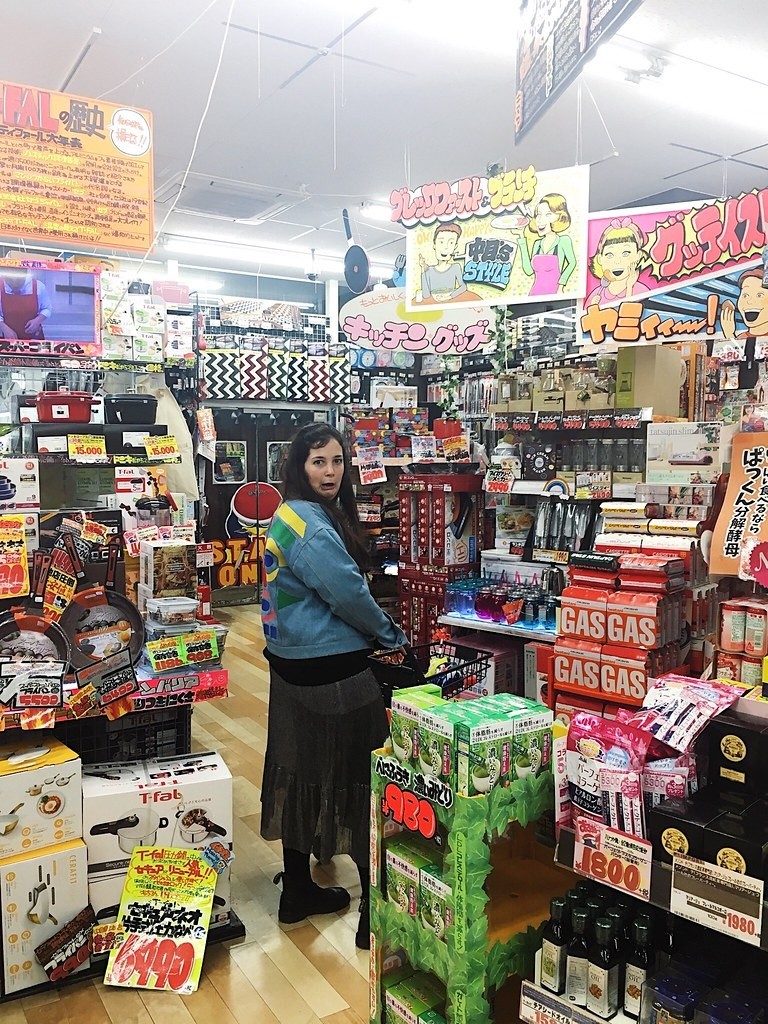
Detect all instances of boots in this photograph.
[355,897,370,950]
[273,864,351,924]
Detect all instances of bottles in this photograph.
[559,439,645,472]
[646,562,719,680]
[541,879,669,1022]
[443,577,562,631]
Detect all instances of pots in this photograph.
[174,808,227,843]
[90,807,168,855]
[135,495,173,529]
[25,773,76,797]
[1,532,145,701]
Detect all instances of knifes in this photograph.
[532,501,592,551]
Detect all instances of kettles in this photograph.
[541,562,565,596]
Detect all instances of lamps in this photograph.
[359,199,394,222]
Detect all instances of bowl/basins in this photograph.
[420,906,445,940]
[514,748,541,779]
[418,746,442,778]
[471,758,500,794]
[386,882,408,914]
[393,731,413,762]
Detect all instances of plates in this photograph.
[35,790,65,819]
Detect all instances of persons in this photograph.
[0,266,52,340]
[259,421,413,952]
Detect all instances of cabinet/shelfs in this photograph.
[351,402,444,600]
[432,408,767,1024]
[198,394,342,608]
[0,289,246,1002]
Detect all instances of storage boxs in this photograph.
[0,250,768,1024]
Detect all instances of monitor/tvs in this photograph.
[0,257,103,357]
